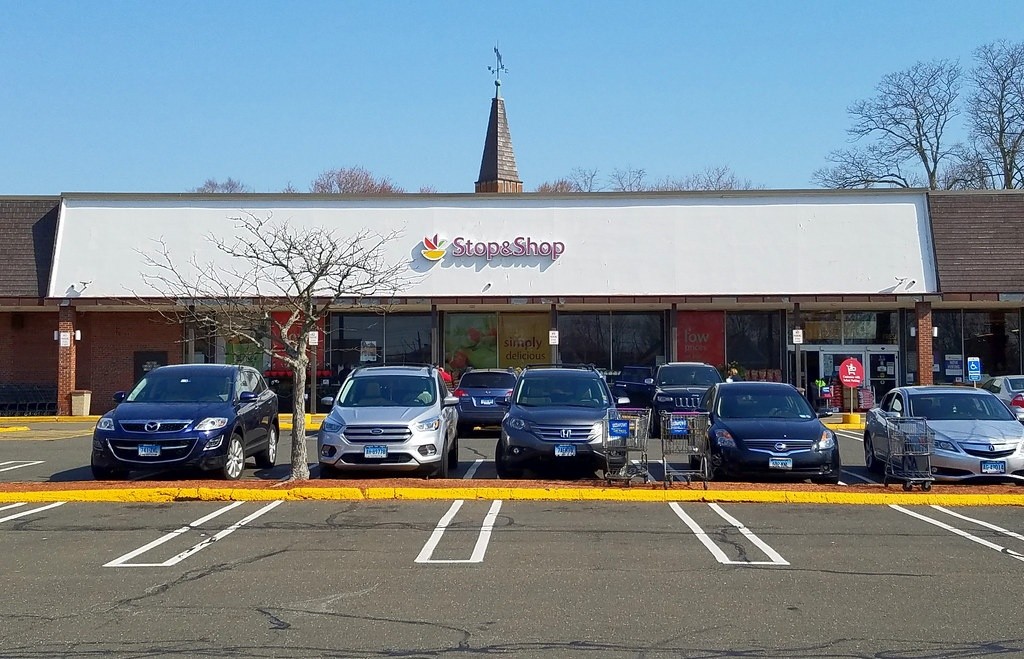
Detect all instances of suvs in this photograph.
[613,362,724,439]
[453,367,517,433]
[496,363,631,480]
[318,362,459,479]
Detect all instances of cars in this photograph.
[90,364,280,481]
[688,382,840,484]
[980,375,1024,424]
[864,386,1024,486]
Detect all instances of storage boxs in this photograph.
[816,376,873,408]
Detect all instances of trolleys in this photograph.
[602,408,651,487]
[660,412,712,490]
[884,417,935,492]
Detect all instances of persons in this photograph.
[730,368,742,381]
[515,366,524,377]
[431,362,452,384]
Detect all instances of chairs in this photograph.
[762,391,787,414]
[401,381,425,402]
[524,380,552,403]
[570,384,600,405]
[694,371,707,383]
[160,378,191,401]
[359,382,385,405]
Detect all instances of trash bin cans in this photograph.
[70,389,92,416]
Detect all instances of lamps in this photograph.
[931,326,938,337]
[910,327,918,336]
